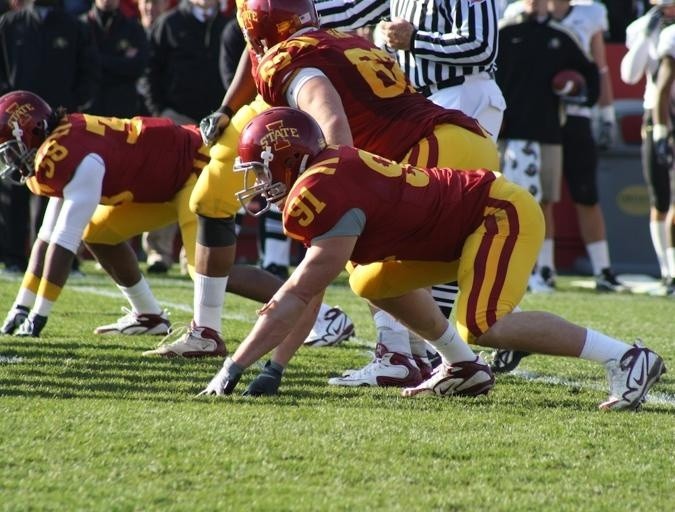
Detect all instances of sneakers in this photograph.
[597,269,625,293]
[95,306,227,357]
[304,306,531,396]
[598,343,666,411]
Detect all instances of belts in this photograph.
[424,76,464,96]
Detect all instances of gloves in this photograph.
[3,305,48,336]
[200,106,232,147]
[195,358,283,395]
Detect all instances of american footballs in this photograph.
[553,70,585,95]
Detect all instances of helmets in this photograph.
[0,91,53,187]
[233,107,326,217]
[237,0,319,56]
